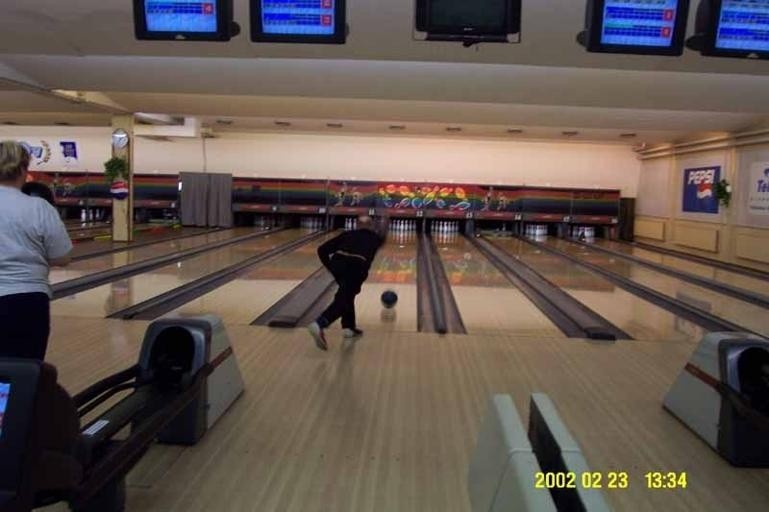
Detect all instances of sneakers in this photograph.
[342,327,362,338]
[309,322,328,351]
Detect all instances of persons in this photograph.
[308,210,393,352]
[0,138,75,361]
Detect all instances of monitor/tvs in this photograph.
[249,1,349,44]
[413,1,521,43]
[687,0,769,59]
[577,0,691,57]
[133,0,240,41]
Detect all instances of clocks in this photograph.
[112,128,129,149]
